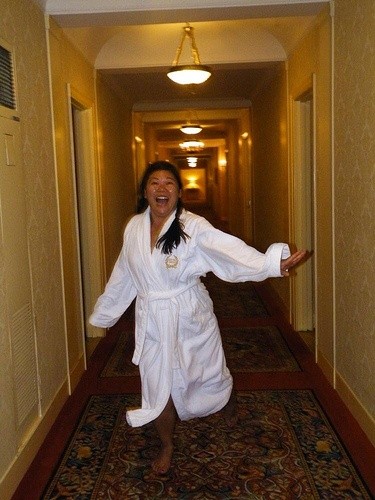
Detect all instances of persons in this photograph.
[87,160,308,475]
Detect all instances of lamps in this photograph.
[166,24,213,167]
